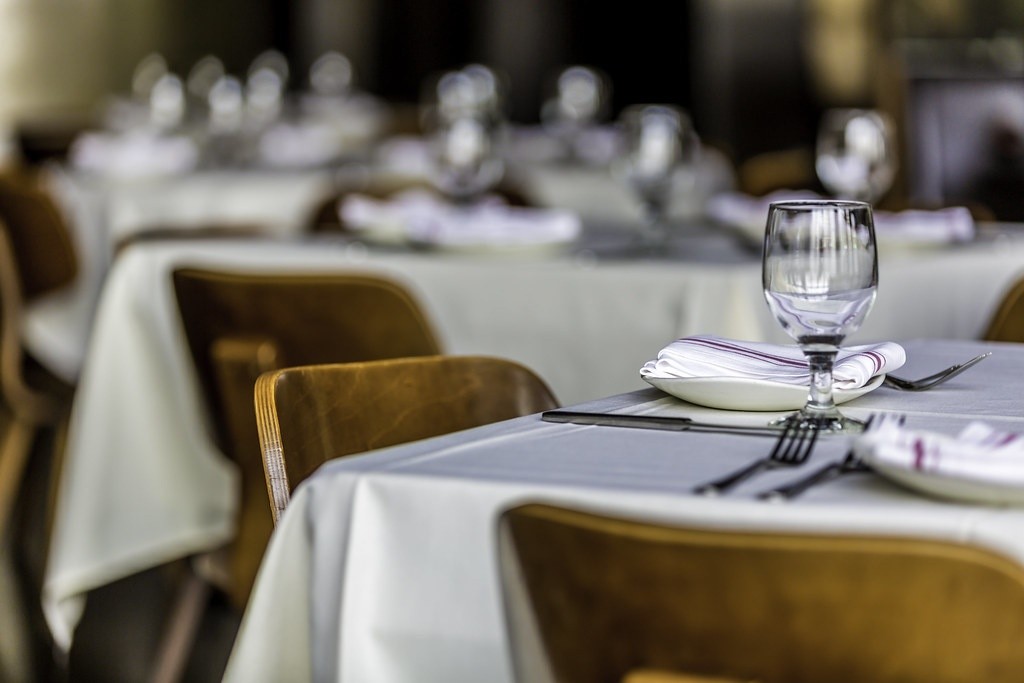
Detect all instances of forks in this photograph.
[754,412,905,503]
[693,412,824,496]
[883,351,990,391]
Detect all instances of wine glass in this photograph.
[609,107,685,258]
[761,196,879,435]
[816,107,898,251]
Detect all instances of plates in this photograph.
[856,439,1024,504]
[640,374,886,411]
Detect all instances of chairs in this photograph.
[2,117,1024,683]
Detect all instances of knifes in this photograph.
[541,411,785,436]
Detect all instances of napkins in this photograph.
[638,336,905,391]
[712,188,973,251]
[857,422,1024,480]
[338,189,585,243]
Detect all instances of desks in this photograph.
[43,218,1024,683]
[30,131,727,381]
[210,338,1024,683]
